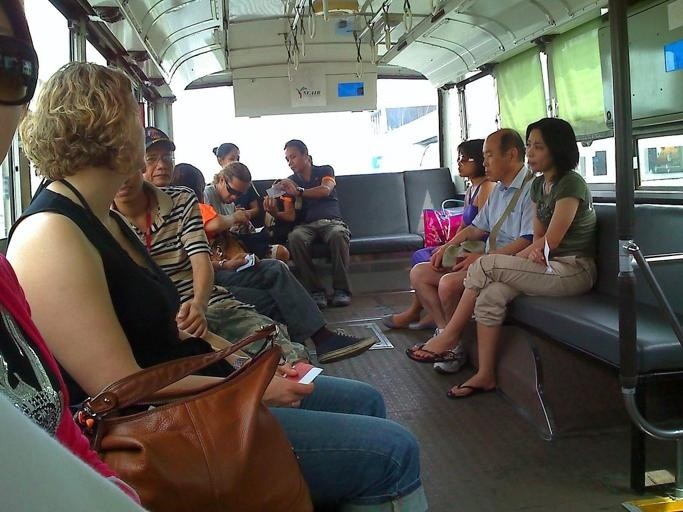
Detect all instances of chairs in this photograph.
[403,167,459,248]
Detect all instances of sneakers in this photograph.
[312,288,497,398]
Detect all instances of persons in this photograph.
[4,62,427,511]
[112,130,377,365]
[406,117,598,399]
[409,127,537,374]
[1,0,148,511]
[380,138,498,331]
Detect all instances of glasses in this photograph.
[458,156,472,162]
[1,34,39,105]
[225,181,243,196]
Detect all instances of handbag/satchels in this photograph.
[442,240,485,269]
[76,322,313,512]
[423,199,465,247]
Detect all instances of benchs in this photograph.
[507,202,683,493]
[251,168,424,258]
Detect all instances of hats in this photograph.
[145,127,175,151]
